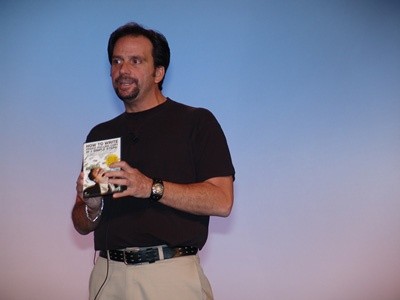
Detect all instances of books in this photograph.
[82,137,121,198]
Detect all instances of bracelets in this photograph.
[85,199,105,222]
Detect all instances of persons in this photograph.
[71,22,236,300]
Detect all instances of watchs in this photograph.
[149,178,165,203]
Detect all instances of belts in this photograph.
[96,246,196,264]
